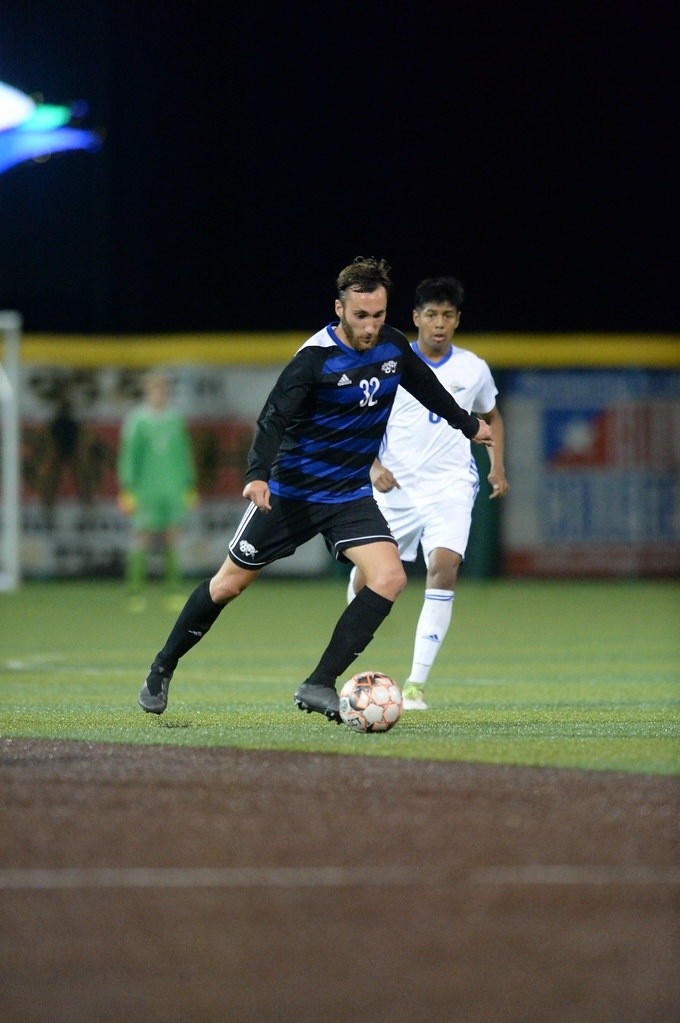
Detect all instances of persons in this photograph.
[346,279,511,712]
[116,370,201,612]
[35,364,102,531]
[139,264,495,724]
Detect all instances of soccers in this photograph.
[338,671,404,735]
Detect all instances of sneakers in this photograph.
[293,679,343,725]
[402,679,428,710]
[138,652,174,715]
